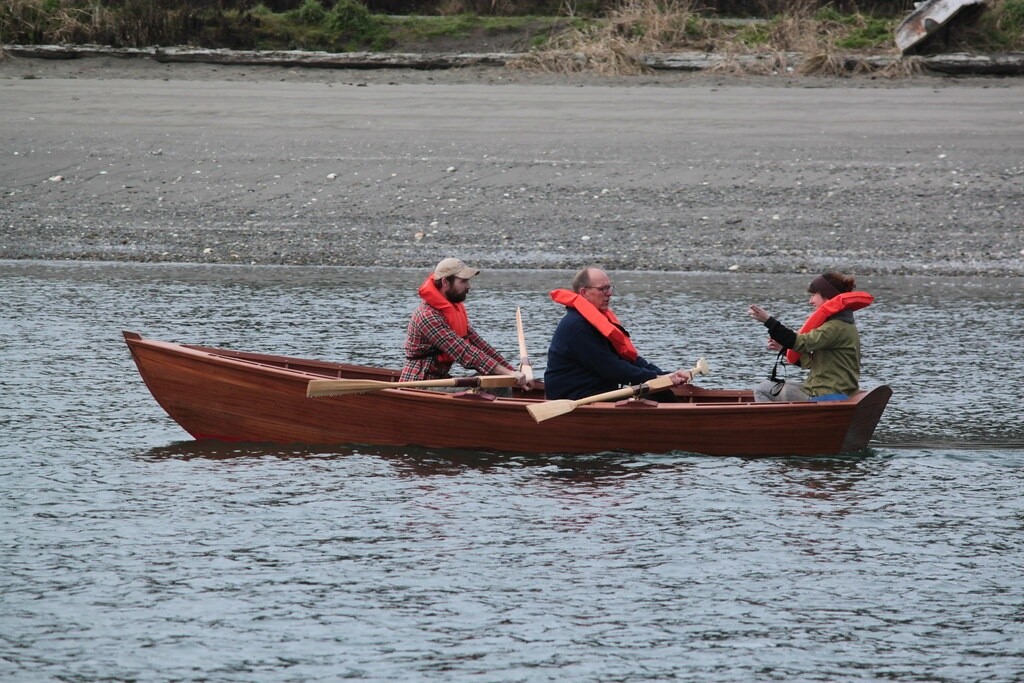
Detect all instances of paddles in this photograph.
[306,373,525,398]
[685,357,709,382]
[515,305,535,389]
[526,374,674,425]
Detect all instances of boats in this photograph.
[122,329,897,458]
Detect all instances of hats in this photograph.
[434,257,480,281]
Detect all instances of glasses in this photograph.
[586,285,615,293]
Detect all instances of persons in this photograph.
[542,266,691,403]
[749,272,861,403]
[396,258,528,398]
